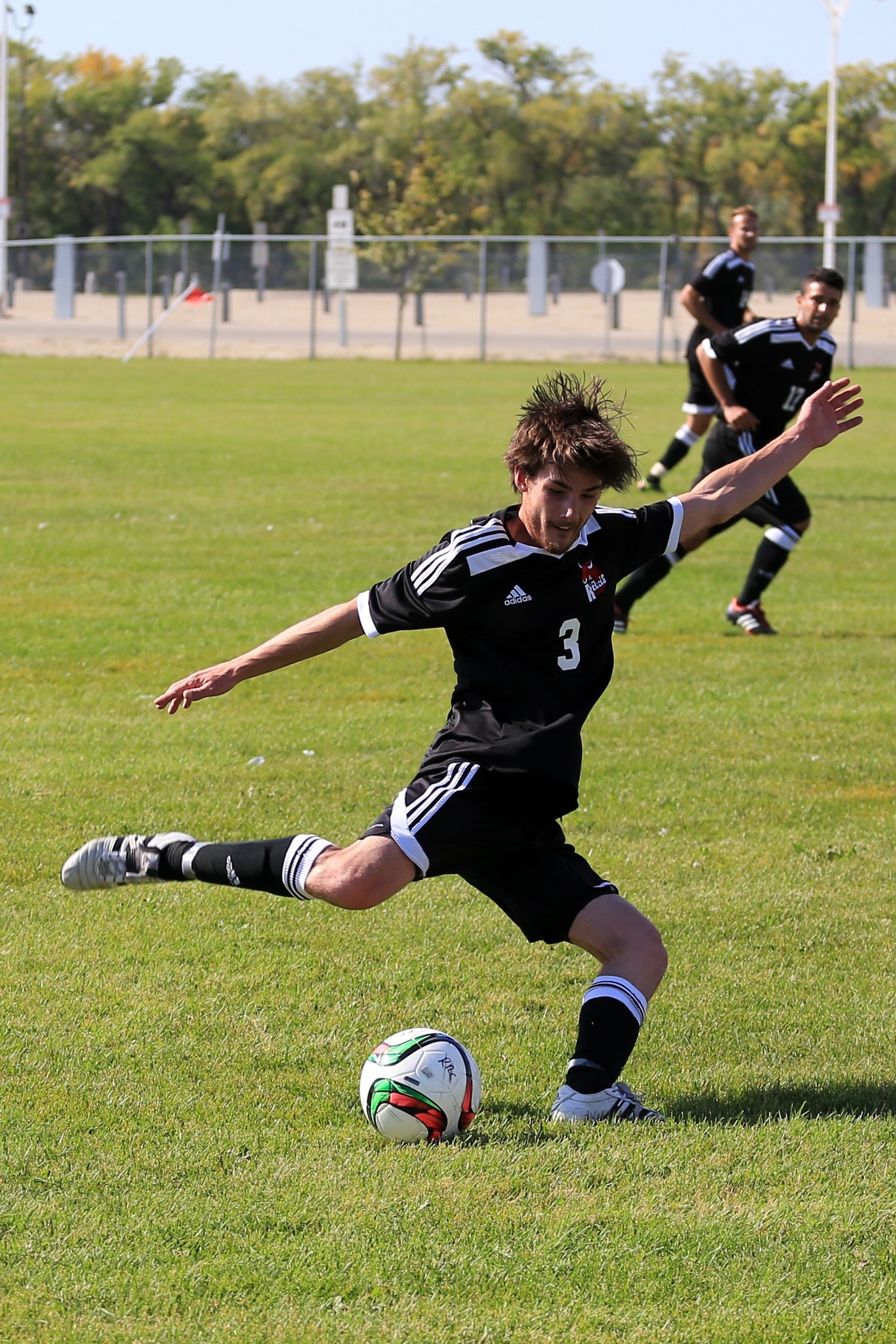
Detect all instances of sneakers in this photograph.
[613,603,627,633]
[550,1081,668,1124]
[726,597,778,635]
[59,831,195,889]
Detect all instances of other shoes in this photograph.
[638,478,669,493]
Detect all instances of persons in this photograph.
[613,268,849,635]
[55,378,867,1121]
[638,206,760,491]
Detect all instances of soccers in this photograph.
[357,1028,476,1150]
